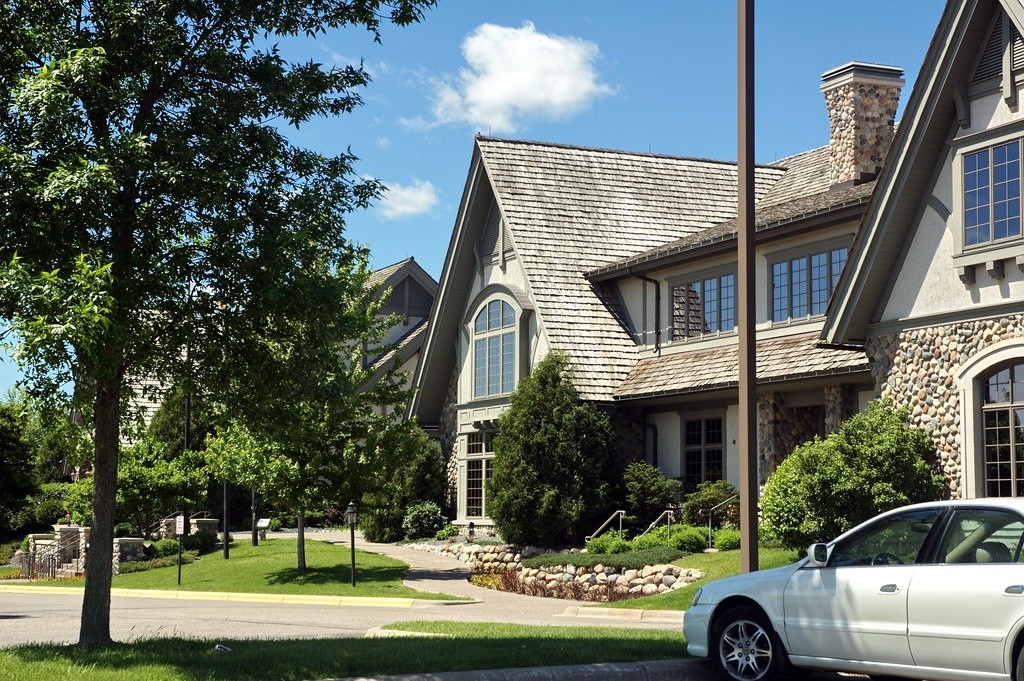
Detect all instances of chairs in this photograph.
[976,541,1011,562]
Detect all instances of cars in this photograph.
[682,497,1024,681]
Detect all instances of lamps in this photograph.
[347,500,357,523]
